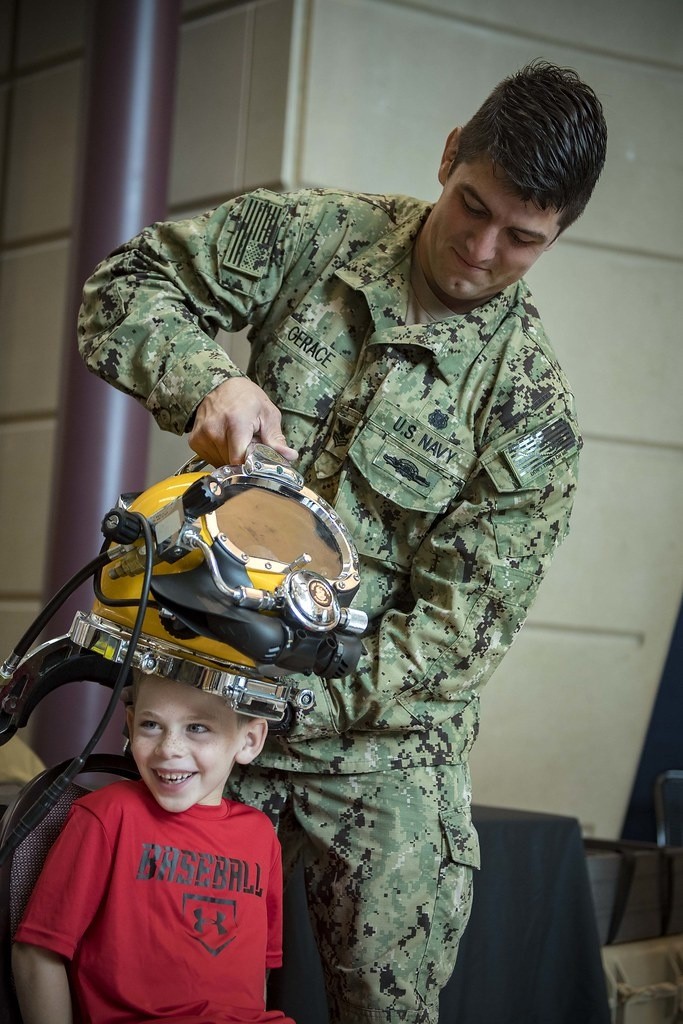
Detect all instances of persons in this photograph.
[77,62,607,1024]
[11,667,296,1024]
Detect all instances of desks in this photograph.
[270,802,613,1024]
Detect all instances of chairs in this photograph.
[653,770,683,847]
[0,754,144,1024]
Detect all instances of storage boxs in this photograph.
[579,839,683,947]
[601,933,683,1024]
